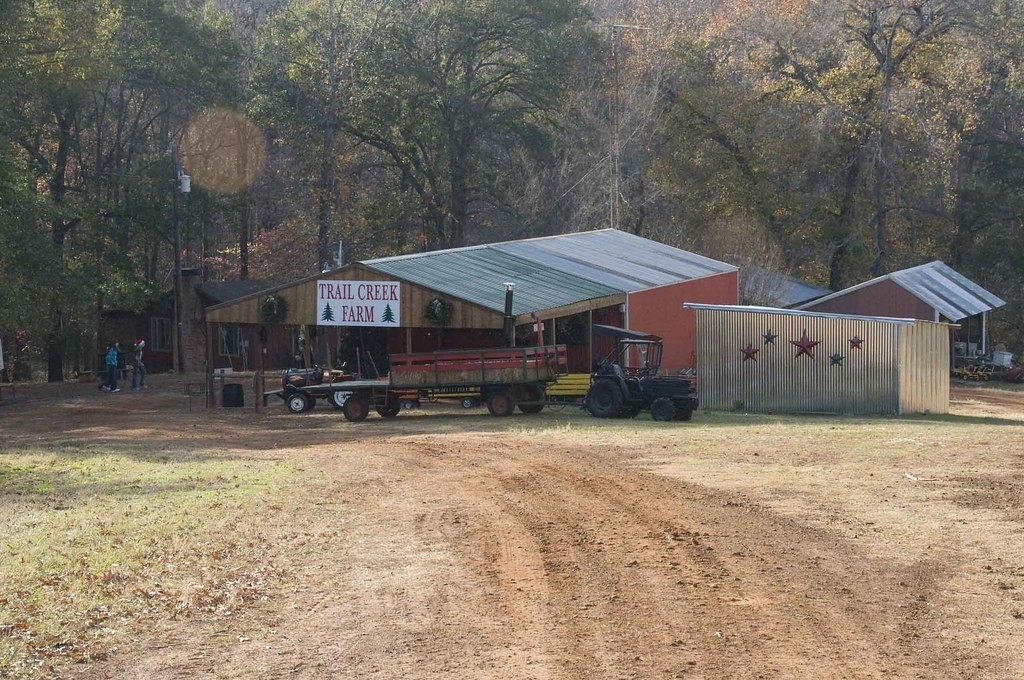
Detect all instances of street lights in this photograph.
[171,170,191,371]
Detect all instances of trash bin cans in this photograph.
[223,383,244,407]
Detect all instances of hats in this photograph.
[134,338,144,346]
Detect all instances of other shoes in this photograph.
[111,389,120,392]
[103,385,111,390]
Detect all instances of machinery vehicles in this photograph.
[583,338,699,421]
[265,361,366,411]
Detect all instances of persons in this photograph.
[100,339,121,392]
[131,338,148,391]
